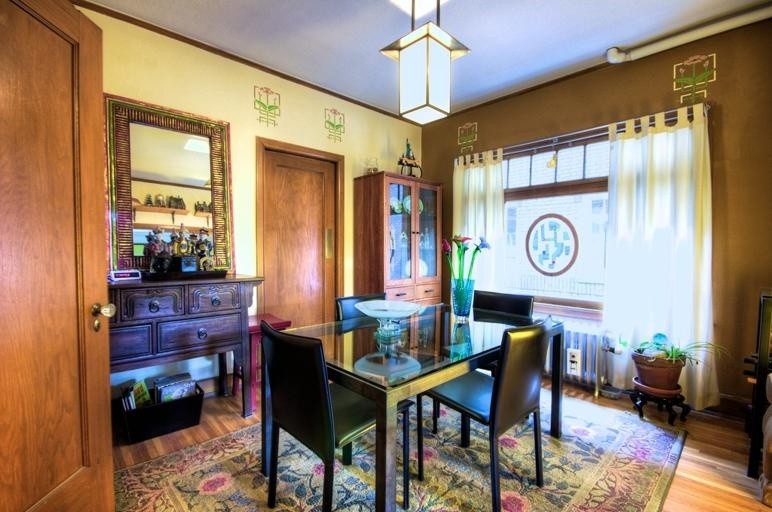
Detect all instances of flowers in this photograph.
[442,235,490,312]
[324,110,345,142]
[458,123,476,152]
[253,85,280,125]
[673,55,716,103]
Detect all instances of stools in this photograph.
[232,315,291,412]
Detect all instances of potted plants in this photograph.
[632,339,733,399]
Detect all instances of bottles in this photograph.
[424,227,429,248]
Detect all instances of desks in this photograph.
[261,302,564,512]
[108,273,266,418]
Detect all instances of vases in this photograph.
[449,320,473,364]
[451,278,475,316]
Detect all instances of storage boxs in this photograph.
[111,383,204,445]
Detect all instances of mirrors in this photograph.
[103,92,237,273]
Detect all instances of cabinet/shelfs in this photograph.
[353,170,444,365]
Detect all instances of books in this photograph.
[120,372,196,411]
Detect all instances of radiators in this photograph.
[546,328,623,398]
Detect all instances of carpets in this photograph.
[113,367,688,512]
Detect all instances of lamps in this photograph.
[380,0,470,126]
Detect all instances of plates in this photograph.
[404,258,428,277]
[403,195,423,216]
[390,197,404,214]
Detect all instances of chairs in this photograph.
[261,321,415,512]
[417,326,547,512]
[335,293,386,322]
[433,290,534,419]
